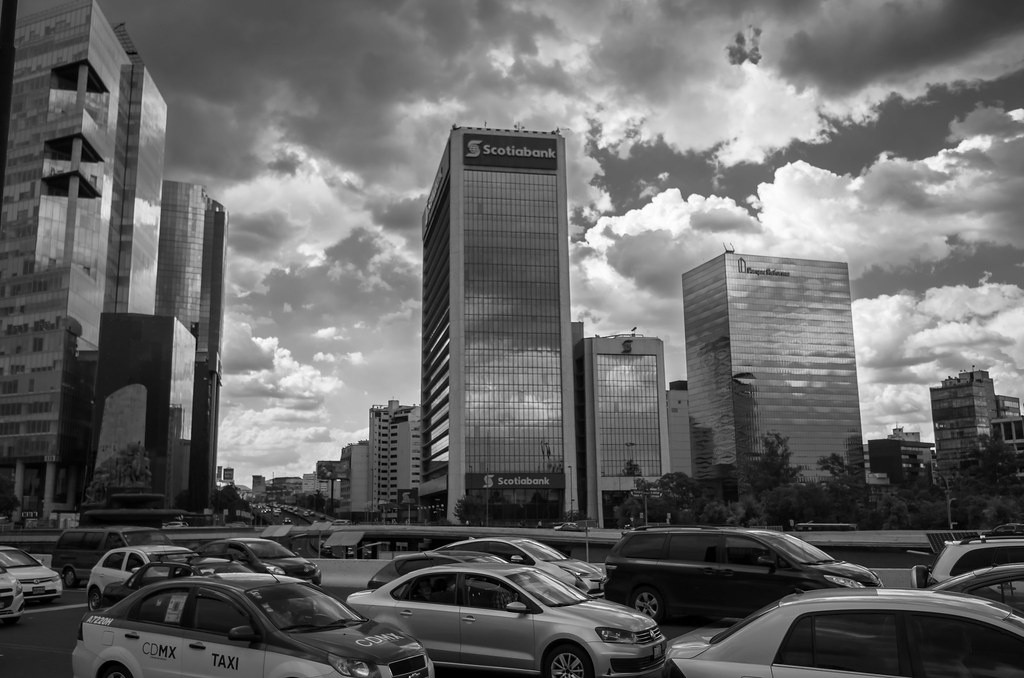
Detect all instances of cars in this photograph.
[0,564,26,627]
[87,544,253,613]
[345,563,668,678]
[926,563,1024,613]
[436,535,609,598]
[0,545,64,605]
[71,572,436,677]
[248,501,325,525]
[365,549,508,592]
[161,521,189,529]
[333,519,352,526]
[195,537,322,587]
[661,586,1024,678]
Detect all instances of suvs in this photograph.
[51,524,174,589]
[910,535,1024,604]
[990,522,1024,536]
[554,522,578,532]
[604,522,884,628]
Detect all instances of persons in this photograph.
[414,581,444,605]
[492,575,521,611]
[752,543,788,567]
[537,519,543,529]
[464,520,471,526]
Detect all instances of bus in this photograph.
[792,522,860,532]
